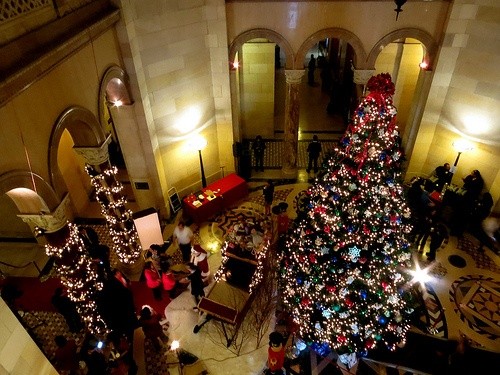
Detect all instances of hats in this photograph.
[191,243,208,255]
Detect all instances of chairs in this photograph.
[167,186,182,213]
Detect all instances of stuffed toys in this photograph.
[232,218,263,246]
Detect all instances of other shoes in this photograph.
[426,252,430,257]
[427,257,433,261]
[419,250,423,253]
[192,306,198,310]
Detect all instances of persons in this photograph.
[252,135,323,245]
[404,163,494,261]
[44,222,210,375]
[307,53,317,84]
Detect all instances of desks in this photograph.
[194,296,238,348]
[182,173,249,224]
